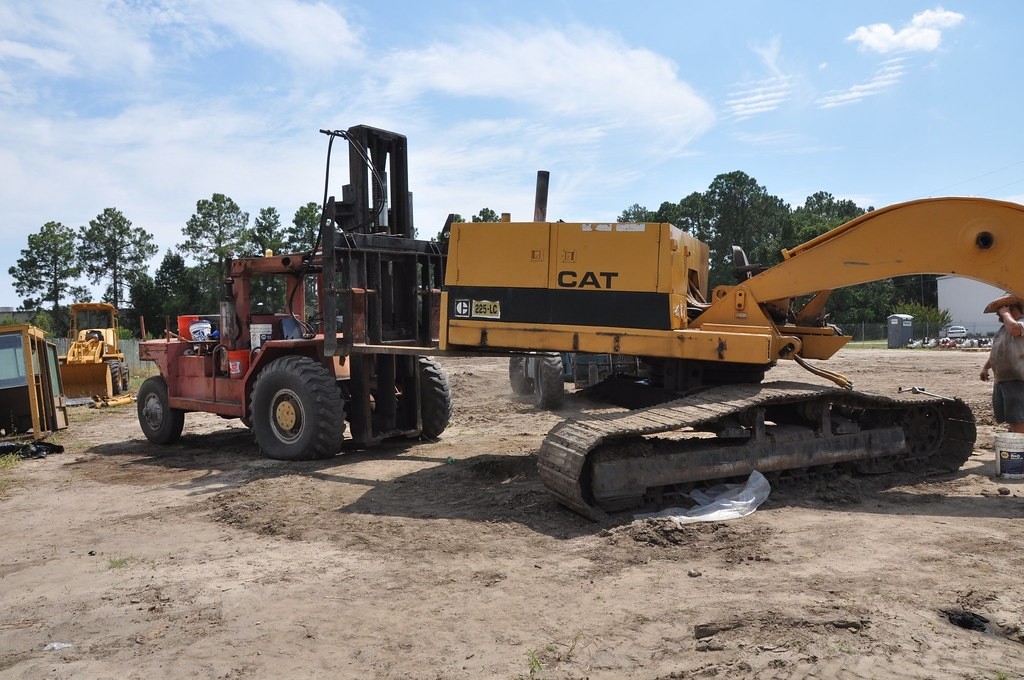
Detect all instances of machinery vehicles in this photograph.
[437,196,1023,524]
[59,303,130,407]
[136,124,559,464]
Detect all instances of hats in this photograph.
[983,292,1024,313]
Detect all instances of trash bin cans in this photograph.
[887,314,914,349]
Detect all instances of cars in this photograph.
[947,324,969,339]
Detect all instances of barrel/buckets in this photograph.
[189,320,212,352]
[250,324,272,356]
[993,431,1024,480]
[177,315,199,341]
[226,349,249,379]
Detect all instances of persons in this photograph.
[980,291,1024,433]
[208,324,220,337]
